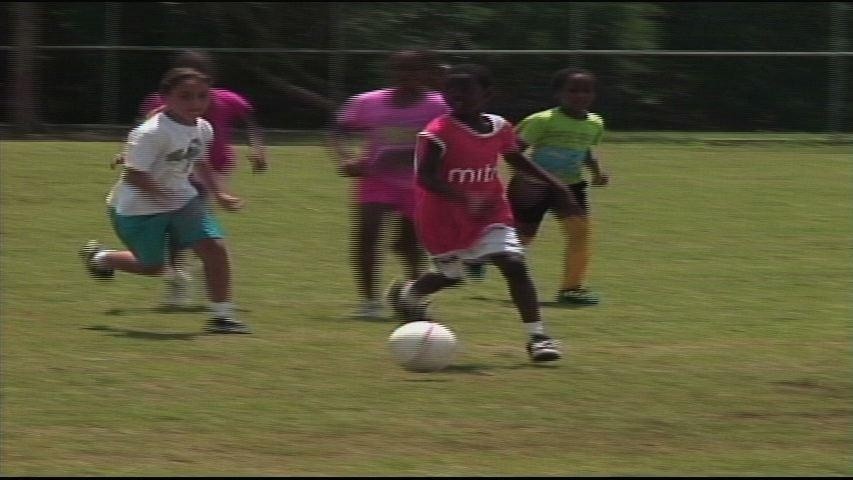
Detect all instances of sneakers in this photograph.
[527,331,561,362]
[383,274,430,320]
[203,311,250,334]
[79,239,113,281]
[558,284,599,305]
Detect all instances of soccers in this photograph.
[388,321,459,373]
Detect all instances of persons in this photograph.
[463,66,609,304]
[325,47,454,311]
[108,51,267,308]
[399,64,577,361]
[80,67,251,335]
